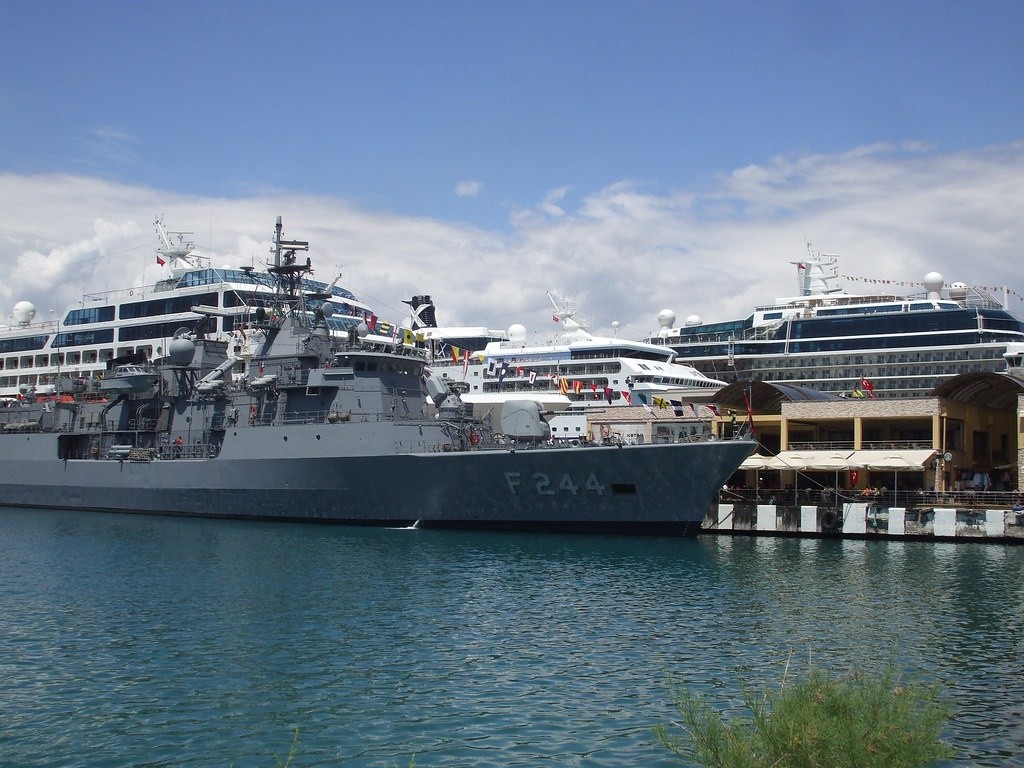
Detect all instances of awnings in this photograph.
[737,449,939,472]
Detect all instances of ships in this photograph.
[630,246,1023,395]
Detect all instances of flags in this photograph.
[743,393,758,434]
[157,257,165,266]
[705,405,723,418]
[798,263,807,270]
[360,309,538,385]
[553,377,701,417]
[862,378,873,390]
[553,316,558,323]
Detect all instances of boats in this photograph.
[400,290,724,409]
[1,213,762,540]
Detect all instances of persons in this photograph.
[175,436,183,458]
[678,426,708,439]
[852,386,863,397]
[867,390,877,399]
[860,485,887,497]
[616,433,623,450]
[821,485,844,504]
[973,473,991,491]
[722,481,814,502]
[916,487,936,504]
[1002,472,1012,491]
